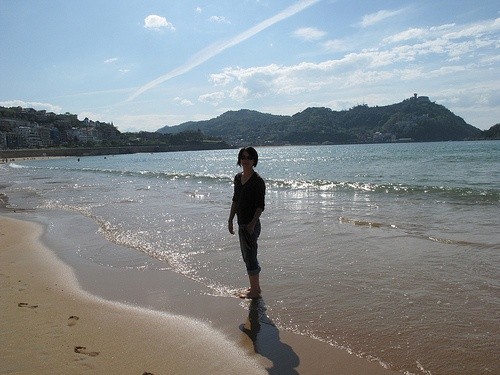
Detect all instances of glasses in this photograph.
[240,155,254,160]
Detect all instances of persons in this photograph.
[228,145,266,299]
